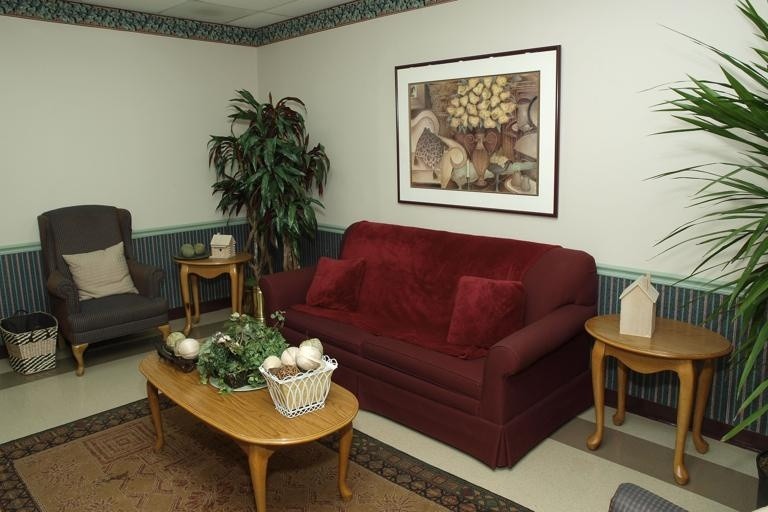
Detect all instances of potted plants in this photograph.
[643,0,768,509]
[208,89,330,323]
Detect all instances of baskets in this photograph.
[0,309,56,375]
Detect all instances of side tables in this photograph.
[175,252,253,337]
[585,314,735,487]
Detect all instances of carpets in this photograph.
[0,393,533,512]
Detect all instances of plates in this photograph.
[174,254,209,260]
[209,375,268,391]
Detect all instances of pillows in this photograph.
[62,241,140,301]
[306,257,366,312]
[448,277,526,350]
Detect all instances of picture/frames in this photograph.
[393,44,562,218]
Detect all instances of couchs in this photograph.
[259,221,598,471]
[38,205,170,377]
